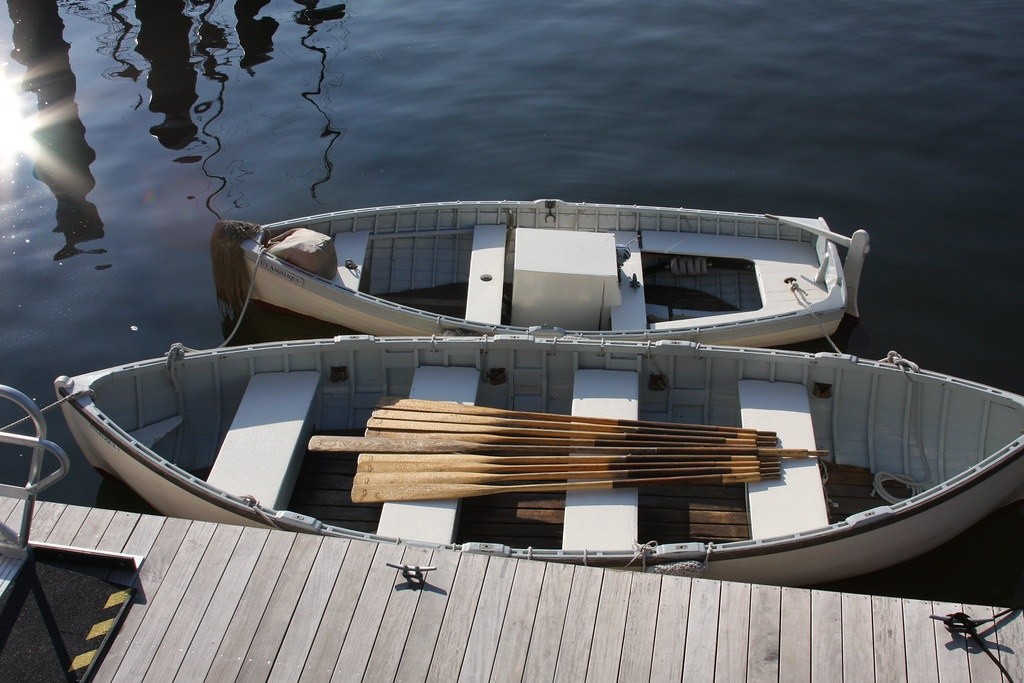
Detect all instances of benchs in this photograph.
[465,224,507,325]
[561,370,639,550]
[607,230,650,331]
[376,363,481,543]
[206,369,321,509]
[737,377,831,540]
[329,231,371,291]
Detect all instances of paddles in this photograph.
[298,396,827,509]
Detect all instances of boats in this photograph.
[205,197,877,352]
[55,331,1024,592]
